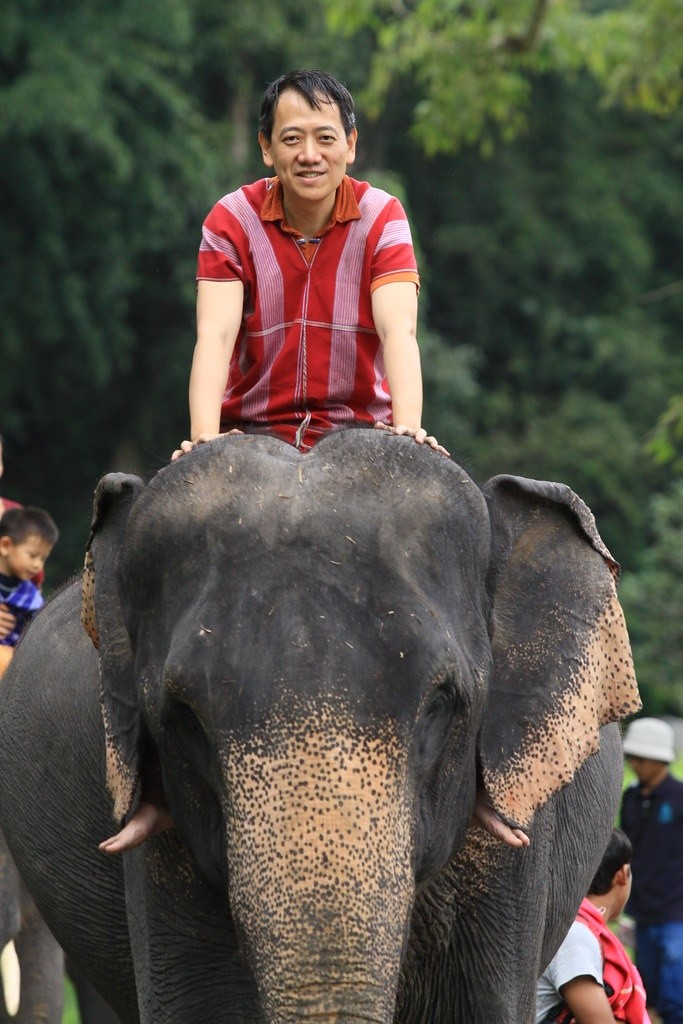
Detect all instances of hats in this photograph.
[623,717,676,763]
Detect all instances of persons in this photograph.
[619,717,683,1024]
[0,435,45,642]
[100,70,530,869]
[0,506,58,678]
[535,828,651,1024]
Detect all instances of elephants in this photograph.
[0,427,646,1024]
